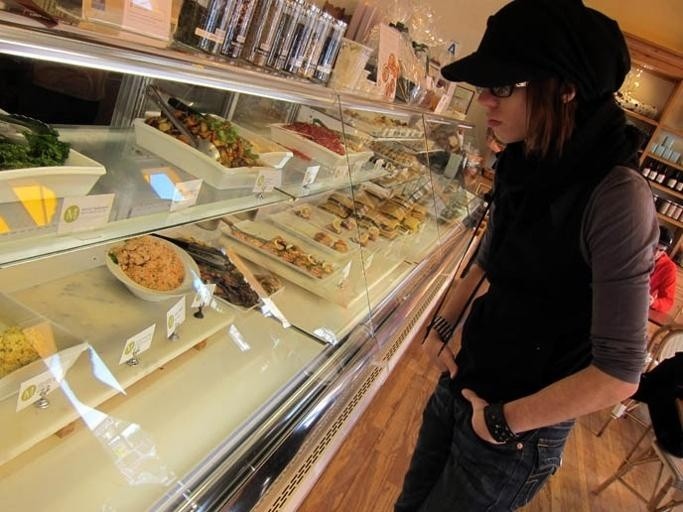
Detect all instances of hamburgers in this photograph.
[317,193,427,239]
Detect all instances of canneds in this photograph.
[652,194,683,223]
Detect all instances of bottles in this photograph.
[640,160,683,196]
[175,0,348,86]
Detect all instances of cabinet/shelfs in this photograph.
[614,27,683,262]
[0,13,486,512]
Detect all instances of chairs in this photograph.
[592,302,682,512]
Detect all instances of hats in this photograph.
[658,226,674,250]
[441,0,630,93]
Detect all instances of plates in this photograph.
[105,233,203,302]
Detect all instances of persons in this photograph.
[389,1,661,511]
[649,226,676,313]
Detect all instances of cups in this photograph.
[326,36,374,93]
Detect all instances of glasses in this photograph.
[475,82,527,98]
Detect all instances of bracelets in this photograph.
[427,316,455,343]
[483,404,519,443]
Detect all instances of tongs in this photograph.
[145,82,222,163]
[312,116,341,142]
[0,112,60,148]
[152,232,236,271]
